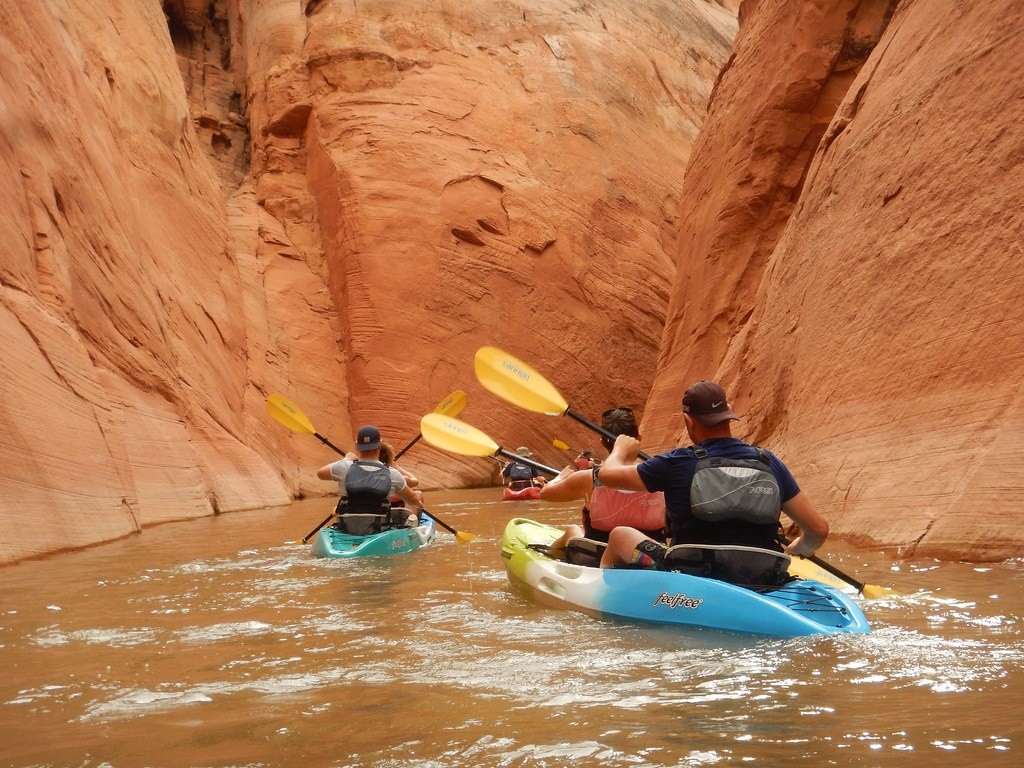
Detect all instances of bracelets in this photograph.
[504,467,506,468]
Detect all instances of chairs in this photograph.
[510,480,534,489]
[334,507,416,534]
[564,537,609,567]
[664,544,791,591]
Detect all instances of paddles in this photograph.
[264,393,479,544]
[293,388,468,544]
[552,440,604,464]
[480,455,548,483]
[472,345,900,599]
[420,412,844,589]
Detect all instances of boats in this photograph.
[311,511,436,560]
[497,515,873,640]
[503,477,547,501]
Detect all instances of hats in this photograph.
[356,426,381,451]
[682,379,740,425]
[515,446,533,457]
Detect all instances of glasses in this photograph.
[601,407,634,418]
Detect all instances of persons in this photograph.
[316,425,424,527]
[496,378,829,574]
